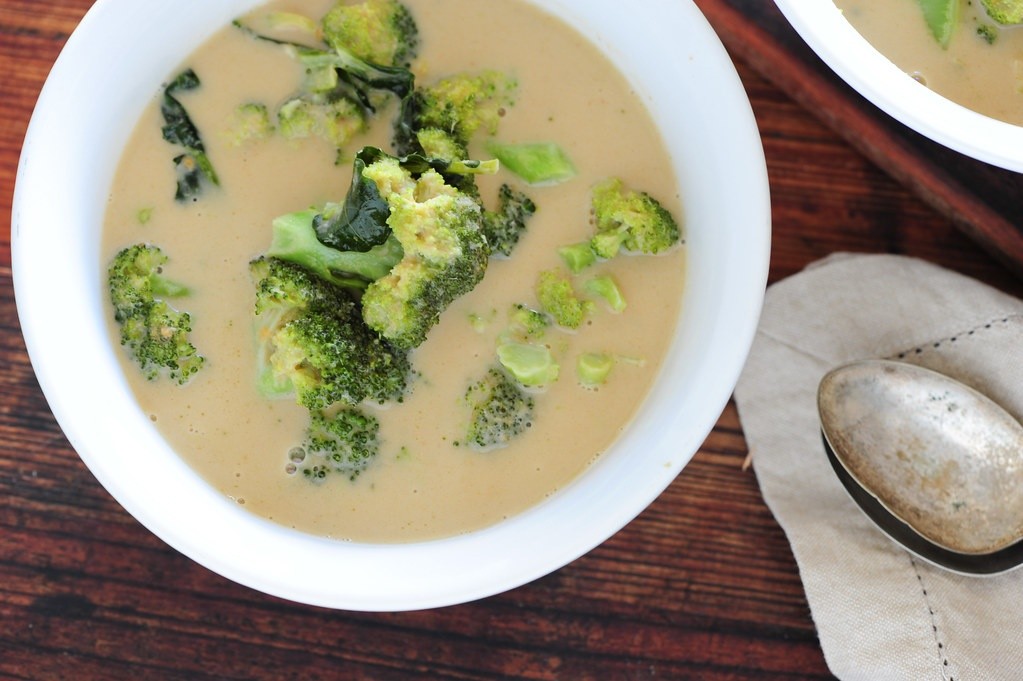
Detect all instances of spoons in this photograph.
[815,357,1023,575]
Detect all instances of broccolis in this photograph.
[980,0,1023,25]
[108,0,680,485]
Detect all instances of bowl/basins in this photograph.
[774,0,1023,175]
[10,0,773,614]
